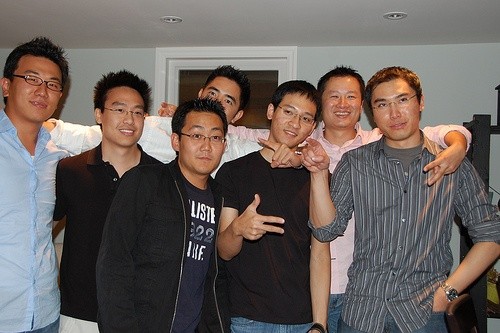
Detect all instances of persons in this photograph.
[213,79,334,332]
[44,64,271,179]
[299,66,500,332]
[295,64,471,332]
[51,70,165,332]
[0,36,178,332]
[95,95,228,331]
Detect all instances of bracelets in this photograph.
[305,322,325,332]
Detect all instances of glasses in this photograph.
[370,93,417,112]
[103,108,149,118]
[177,131,223,144]
[274,103,316,125]
[5,74,63,92]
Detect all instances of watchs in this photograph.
[442,282,461,303]
